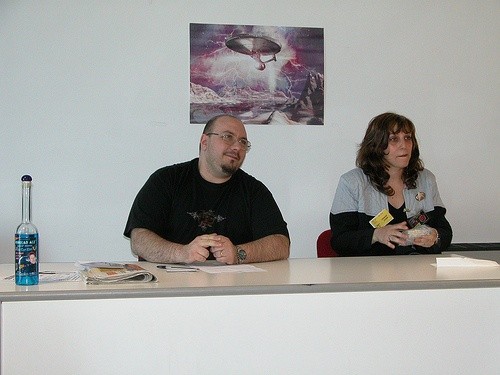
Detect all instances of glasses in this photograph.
[206,133,251,149]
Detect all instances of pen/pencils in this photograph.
[157,266,199,270]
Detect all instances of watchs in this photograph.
[236,244,248,264]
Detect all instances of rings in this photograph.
[388,235,391,242]
[220,250,224,257]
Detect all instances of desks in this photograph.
[1,251,500,374]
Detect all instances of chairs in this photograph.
[317,230,337,257]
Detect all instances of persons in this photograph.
[329,111,454,256]
[122,115,291,265]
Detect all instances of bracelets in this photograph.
[431,228,440,245]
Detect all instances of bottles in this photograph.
[15,174,39,286]
